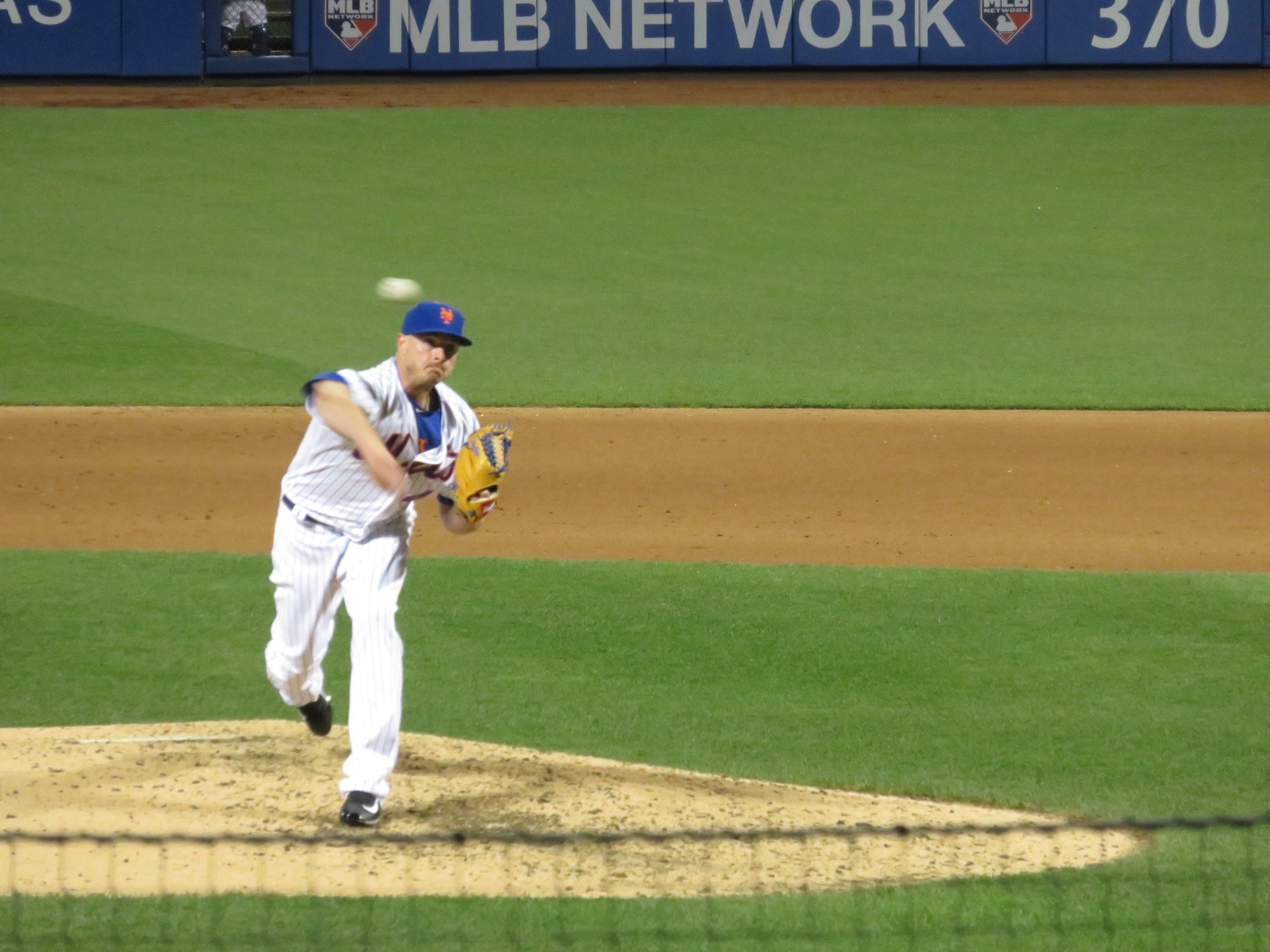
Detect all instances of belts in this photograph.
[282,495,331,526]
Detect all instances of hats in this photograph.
[401,301,473,346]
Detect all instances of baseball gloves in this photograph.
[449,421,515,525]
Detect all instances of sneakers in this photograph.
[298,694,332,736]
[339,791,381,827]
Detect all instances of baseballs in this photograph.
[394,278,419,303]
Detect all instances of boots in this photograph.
[249,23,272,56]
[220,24,235,55]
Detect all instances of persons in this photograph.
[220,0,269,55]
[264,299,516,827]
